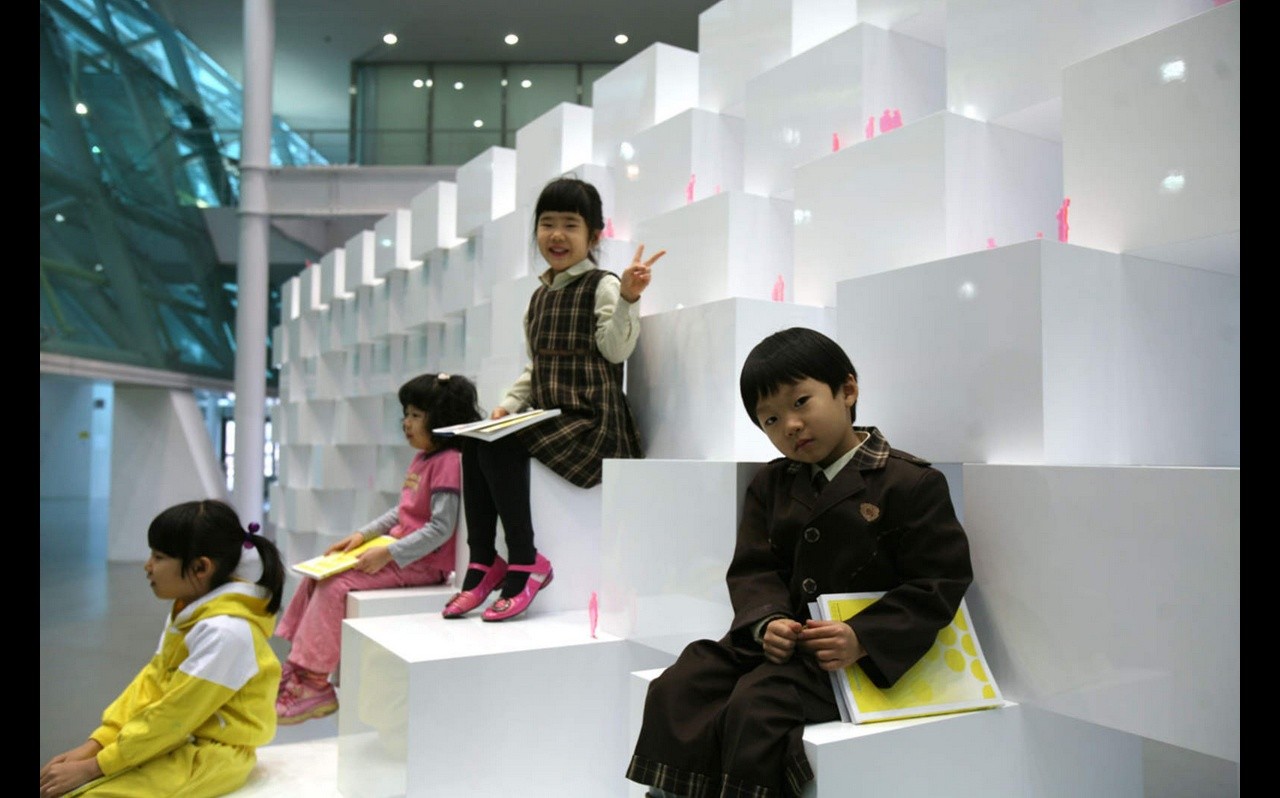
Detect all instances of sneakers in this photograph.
[276,661,340,725]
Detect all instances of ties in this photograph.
[811,470,829,499]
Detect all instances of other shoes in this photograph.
[483,551,553,620]
[442,555,508,617]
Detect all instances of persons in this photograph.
[39,499,285,798]
[273,373,487,725]
[625,328,974,798]
[443,172,666,623]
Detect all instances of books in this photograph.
[291,534,401,580]
[808,591,1005,725]
[433,409,561,442]
[59,736,194,798]
